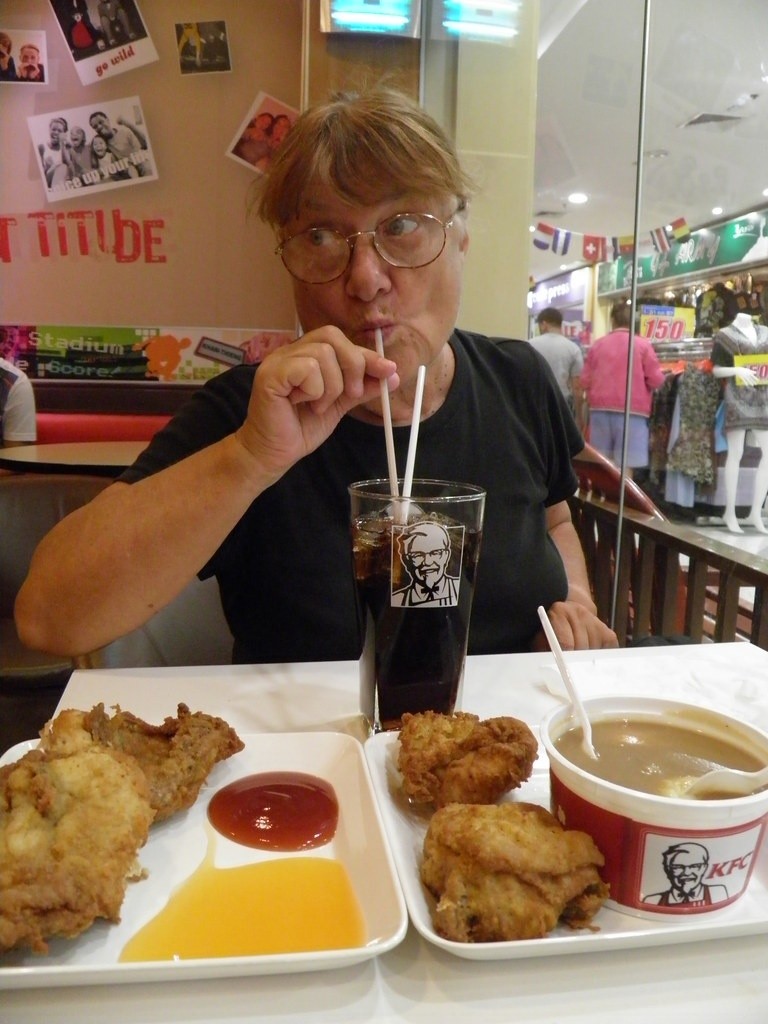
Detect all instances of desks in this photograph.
[1,440,155,478]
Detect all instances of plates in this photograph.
[0,729,401,988]
[375,700,767,959]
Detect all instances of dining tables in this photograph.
[1,642,767,1024]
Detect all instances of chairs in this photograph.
[571,438,768,650]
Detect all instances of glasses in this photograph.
[274,206,465,286]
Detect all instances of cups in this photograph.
[343,479,486,734]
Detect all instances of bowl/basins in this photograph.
[543,696,768,914]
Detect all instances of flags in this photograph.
[533,216,691,265]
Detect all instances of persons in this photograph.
[0,32,18,82]
[90,135,121,181]
[18,43,44,83]
[204,33,228,71]
[241,111,276,167]
[526,308,586,437]
[66,10,103,55]
[64,0,97,39]
[581,301,665,493]
[704,311,767,535]
[89,111,148,178]
[36,118,74,189]
[175,22,204,72]
[263,114,293,169]
[97,0,136,46]
[69,125,98,187]
[0,356,37,448]
[10,79,623,666]
